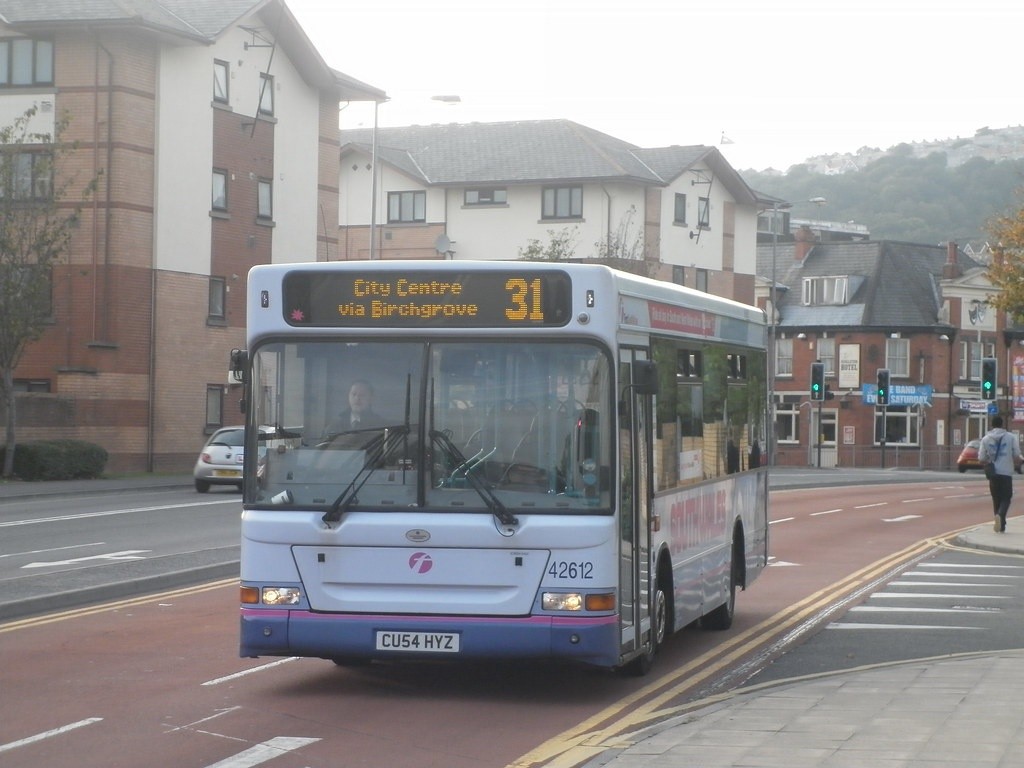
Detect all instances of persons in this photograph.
[321,379,387,456]
[726,440,739,474]
[978,416,1021,533]
[749,439,760,469]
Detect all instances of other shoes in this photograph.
[994,515,1006,532]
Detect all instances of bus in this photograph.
[227,260,772,676]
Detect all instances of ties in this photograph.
[352,419,359,431]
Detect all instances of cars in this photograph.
[193,425,296,492]
[957,439,1023,474]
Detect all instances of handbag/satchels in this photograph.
[984,464,997,480]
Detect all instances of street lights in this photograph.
[770,197,832,467]
[370,92,462,256]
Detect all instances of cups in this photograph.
[271,489,293,504]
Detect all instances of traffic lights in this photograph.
[876,369,889,406]
[812,362,826,401]
[980,358,998,401]
